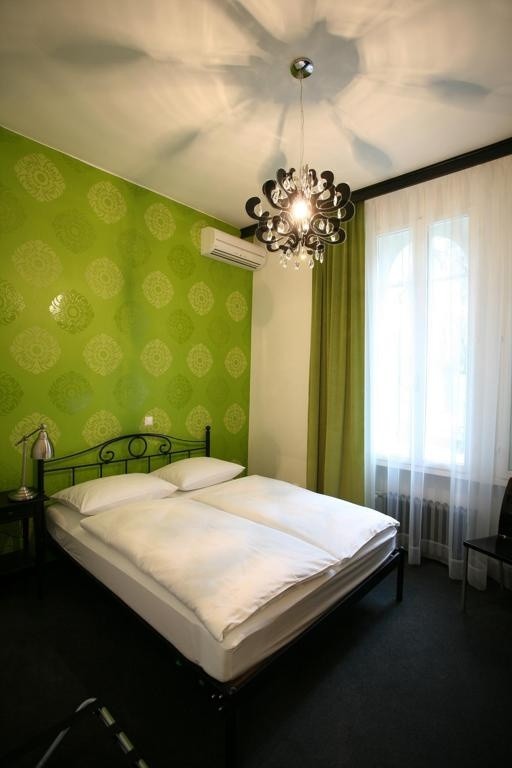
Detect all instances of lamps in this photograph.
[9,423,54,502]
[241,57,355,272]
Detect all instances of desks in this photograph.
[0,487,50,570]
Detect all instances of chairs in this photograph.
[460,477,511,613]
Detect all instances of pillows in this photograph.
[50,456,245,516]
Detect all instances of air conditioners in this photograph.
[199,227,268,272]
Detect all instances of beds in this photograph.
[35,426,408,738]
